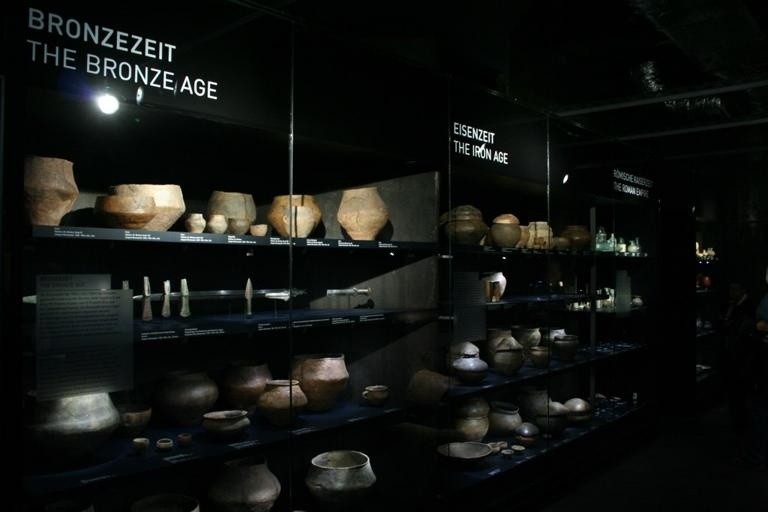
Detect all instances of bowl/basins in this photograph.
[251,223,268,237]
[93,193,159,230]
[437,439,495,470]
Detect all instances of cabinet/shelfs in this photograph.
[0,3,768,512]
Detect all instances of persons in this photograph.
[692,276,758,440]
[744,266,767,473]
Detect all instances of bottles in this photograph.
[183,211,207,234]
[208,214,229,235]
[227,217,250,236]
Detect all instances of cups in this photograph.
[361,384,391,404]
